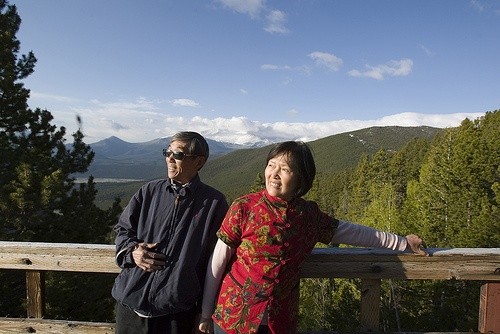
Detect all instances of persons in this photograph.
[196,141,427,334]
[110,131,230,334]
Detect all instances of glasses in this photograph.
[162,149,198,160]
